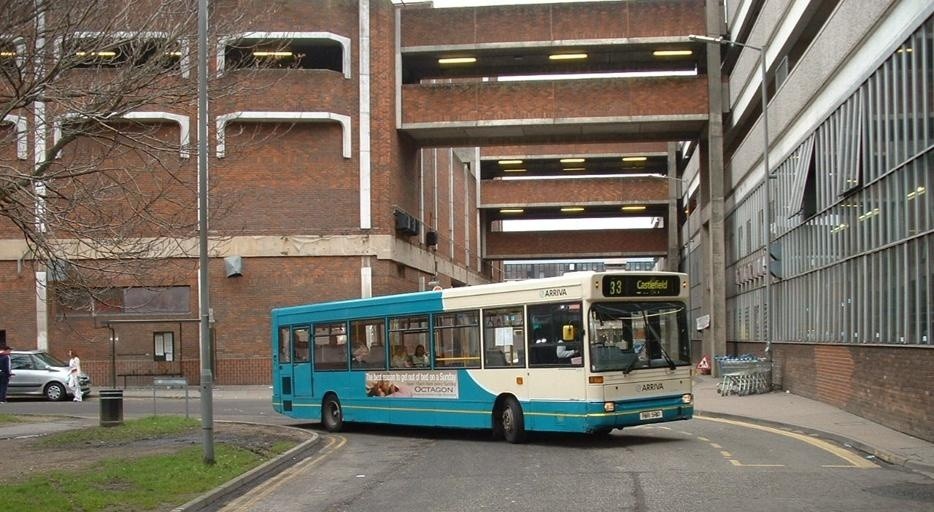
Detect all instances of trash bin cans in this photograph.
[99,390,124,427]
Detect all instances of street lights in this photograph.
[685,30,777,388]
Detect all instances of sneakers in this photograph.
[73,392,83,402]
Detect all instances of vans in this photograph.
[0,349,93,399]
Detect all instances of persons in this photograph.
[392,345,410,368]
[0,347,11,403]
[412,345,430,368]
[68,349,84,401]
[556,325,580,364]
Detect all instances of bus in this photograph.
[262,265,697,442]
